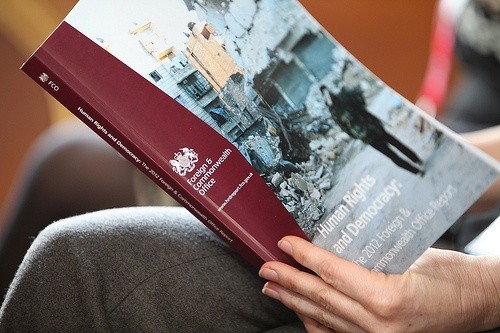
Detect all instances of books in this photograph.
[20,0,500,275]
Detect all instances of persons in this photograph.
[0,112,500,333]
[321,85,425,177]
